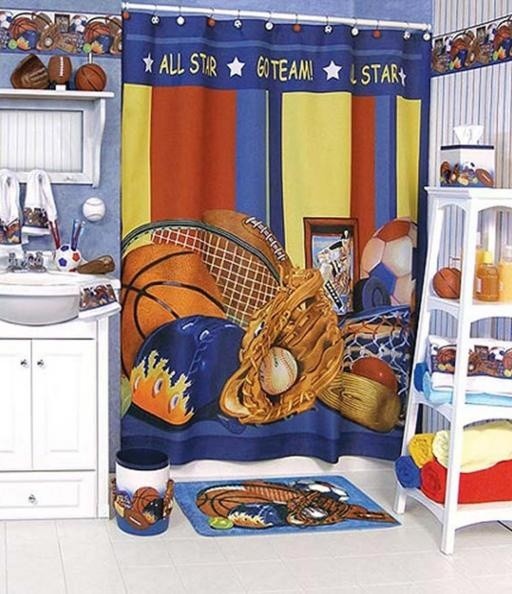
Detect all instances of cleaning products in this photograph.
[475,233,512,301]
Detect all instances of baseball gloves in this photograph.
[219,268,345,424]
[286,490,350,528]
[446,164,460,185]
[77,255,116,274]
[467,352,487,376]
[10,53,50,89]
[36,23,62,52]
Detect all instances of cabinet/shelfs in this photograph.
[392,186,512,557]
[0,316,110,520]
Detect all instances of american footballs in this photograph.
[48,57,71,84]
[123,508,151,531]
[476,168,494,187]
[201,209,295,327]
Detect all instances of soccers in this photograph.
[489,347,506,362]
[360,217,420,317]
[463,160,476,173]
[291,480,350,504]
[53,244,82,272]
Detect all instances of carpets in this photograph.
[172,475,402,537]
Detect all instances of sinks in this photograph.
[0,272,80,325]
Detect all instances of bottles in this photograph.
[472,229,512,304]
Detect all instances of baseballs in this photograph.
[45,38,52,46]
[302,506,329,519]
[259,346,299,396]
[451,174,456,180]
[468,364,474,370]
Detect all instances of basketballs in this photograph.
[83,21,110,44]
[75,64,106,91]
[133,487,160,514]
[440,161,451,176]
[9,17,37,41]
[196,490,272,517]
[434,268,460,299]
[503,351,512,372]
[121,244,224,381]
[435,349,455,362]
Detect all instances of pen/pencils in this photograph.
[49,219,85,250]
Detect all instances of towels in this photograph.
[395,417,511,504]
[0,169,59,247]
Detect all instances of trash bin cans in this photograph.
[114,447,171,536]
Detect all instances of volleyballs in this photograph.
[83,197,105,221]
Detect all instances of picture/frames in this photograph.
[303,217,360,323]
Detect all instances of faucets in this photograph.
[9,259,24,268]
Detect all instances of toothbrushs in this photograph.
[46,218,60,249]
[71,218,85,249]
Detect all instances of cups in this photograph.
[453,125,485,146]
